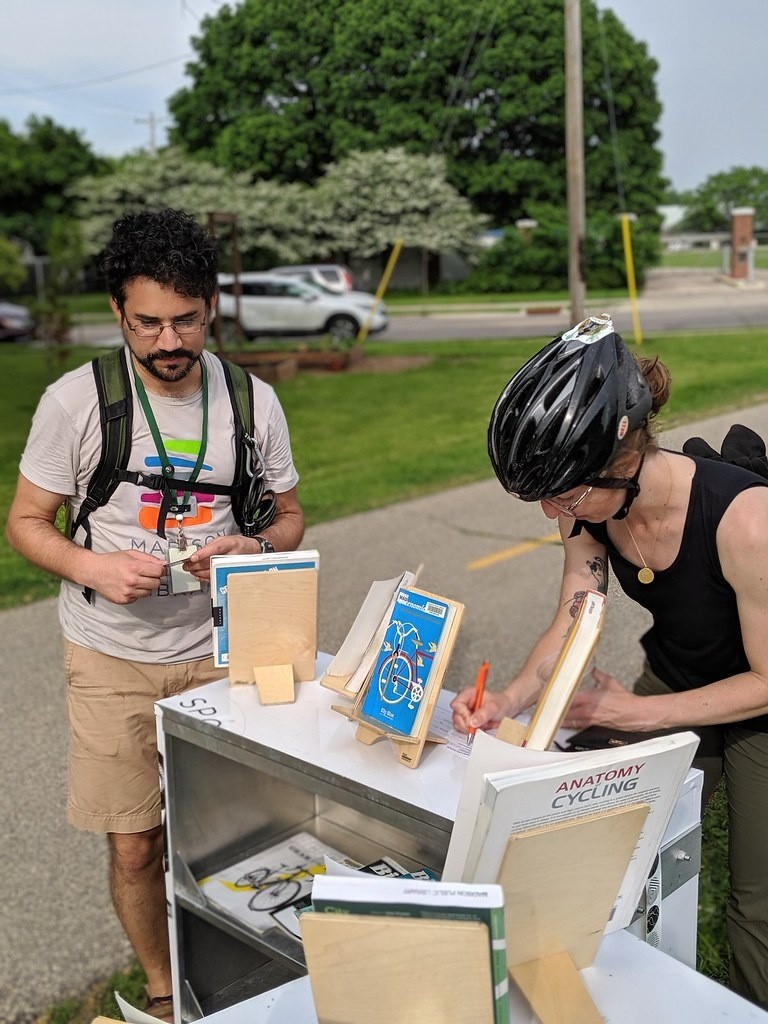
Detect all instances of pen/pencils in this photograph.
[466,657,490,747]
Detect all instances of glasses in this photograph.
[118,296,208,337]
[544,468,611,518]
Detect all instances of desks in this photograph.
[188,928,768,1024]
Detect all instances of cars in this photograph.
[0,302,35,341]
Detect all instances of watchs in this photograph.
[250,537,274,553]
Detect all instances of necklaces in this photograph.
[623,451,673,583]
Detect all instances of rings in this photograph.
[572,720,577,729]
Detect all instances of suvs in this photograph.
[269,265,353,292]
[208,273,389,343]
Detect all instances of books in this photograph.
[440,588,701,971]
[319,571,458,739]
[298,853,511,1024]
[196,830,440,943]
[209,549,320,682]
[90,991,172,1024]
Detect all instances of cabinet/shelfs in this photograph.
[155,651,703,1024]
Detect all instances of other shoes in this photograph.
[146,997,175,1023]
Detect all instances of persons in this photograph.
[5,209,307,1024]
[450,314,768,1012]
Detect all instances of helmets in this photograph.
[487,312,653,503]
